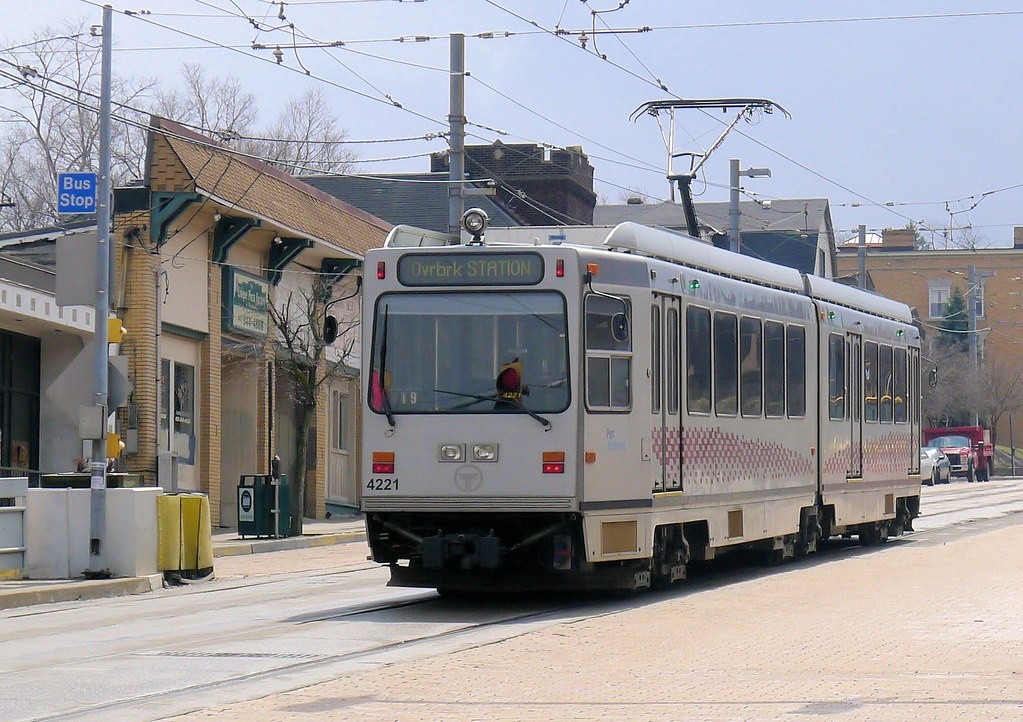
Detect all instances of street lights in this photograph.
[729,159,772,254]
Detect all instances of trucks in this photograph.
[922,425,994,482]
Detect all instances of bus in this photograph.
[358,208,926,603]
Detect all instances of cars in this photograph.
[920,447,951,486]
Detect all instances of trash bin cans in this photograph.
[235,472,291,536]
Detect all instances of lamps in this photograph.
[213,210,221,222]
[491,190,527,222]
[330,264,337,270]
[273,230,282,244]
